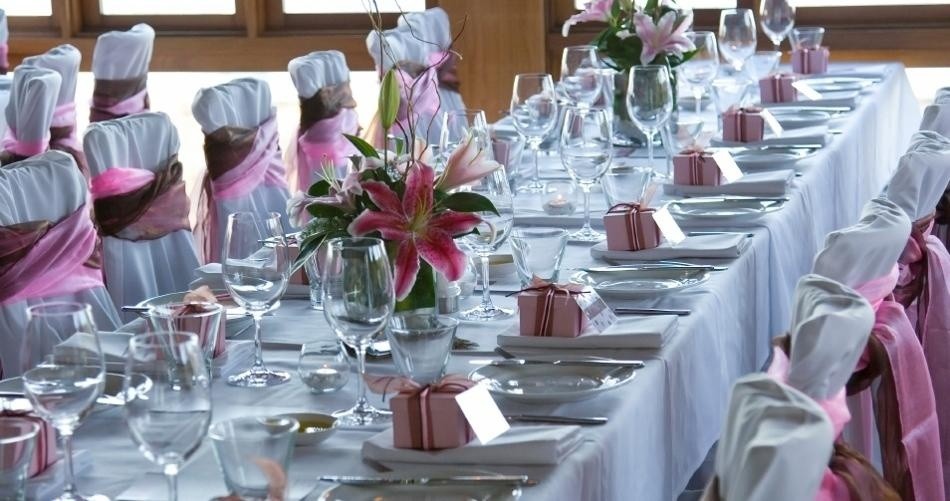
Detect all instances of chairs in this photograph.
[83,22,156,123]
[811,196,949,475]
[1,149,125,376]
[691,367,839,501]
[366,28,450,157]
[765,271,902,500]
[189,75,297,270]
[884,129,949,312]
[0,64,63,166]
[918,82,950,138]
[397,7,469,141]
[286,48,368,205]
[20,42,83,168]
[82,109,200,325]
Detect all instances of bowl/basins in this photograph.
[265,413,336,452]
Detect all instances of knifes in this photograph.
[1,389,128,409]
[487,359,645,370]
[503,412,609,428]
[609,304,691,318]
[663,261,726,274]
[317,473,530,490]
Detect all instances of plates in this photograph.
[318,465,522,501]
[724,145,818,169]
[137,288,281,338]
[800,78,871,95]
[1,371,154,426]
[576,265,713,298]
[759,107,835,128]
[666,194,785,222]
[469,352,637,404]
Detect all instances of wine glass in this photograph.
[442,7,755,214]
[558,106,612,246]
[126,334,213,501]
[19,303,105,501]
[448,161,520,323]
[220,212,289,391]
[321,237,393,433]
[761,0,797,79]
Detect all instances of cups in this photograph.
[505,229,570,290]
[786,27,825,52]
[748,51,782,86]
[299,343,350,395]
[208,414,298,501]
[384,313,458,388]
[1,421,42,500]
[148,301,221,362]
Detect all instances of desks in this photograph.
[413,56,927,349]
[0,220,773,500]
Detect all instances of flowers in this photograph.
[309,135,504,300]
[560,0,704,68]
